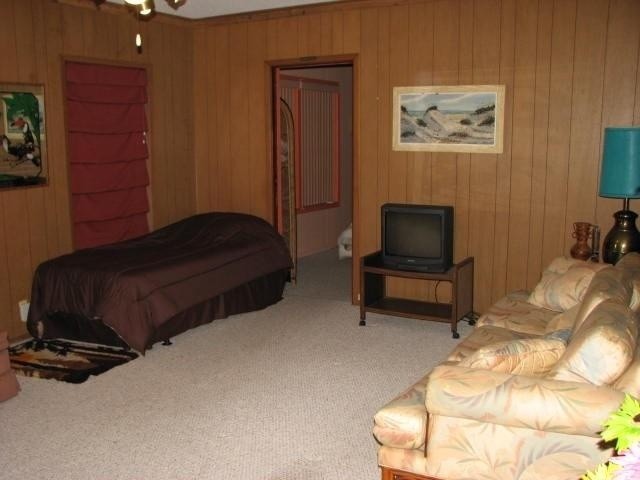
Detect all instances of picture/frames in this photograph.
[392,85,506,154]
[0,81,49,191]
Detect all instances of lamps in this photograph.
[597,127,640,268]
[101,0,187,48]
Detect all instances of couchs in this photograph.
[374,253,640,480]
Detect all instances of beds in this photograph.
[27,212,293,355]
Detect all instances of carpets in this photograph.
[8,336,138,384]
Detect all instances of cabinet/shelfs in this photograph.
[358,250,474,337]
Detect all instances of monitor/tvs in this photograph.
[380,202,454,274]
